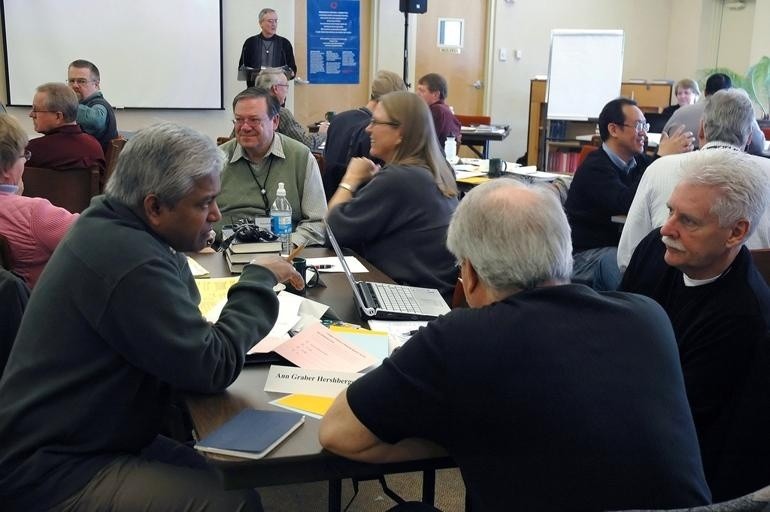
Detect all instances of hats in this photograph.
[371,70,407,97]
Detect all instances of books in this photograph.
[548,120,601,174]
[193,406,306,461]
[222,228,283,273]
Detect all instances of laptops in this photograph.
[322,217,452,320]
[644,113,672,133]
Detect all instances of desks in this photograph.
[450,119,513,157]
[173,216,483,511]
[440,155,579,204]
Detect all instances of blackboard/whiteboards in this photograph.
[544,29,625,122]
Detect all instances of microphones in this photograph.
[242,47,247,69]
[281,48,287,68]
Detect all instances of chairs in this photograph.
[1,236,34,378]
[106,133,128,185]
[453,113,490,130]
[21,155,101,214]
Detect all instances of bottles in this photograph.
[270,181,294,258]
[444,134,458,159]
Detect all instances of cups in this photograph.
[324,111,335,121]
[284,256,318,296]
[488,157,508,179]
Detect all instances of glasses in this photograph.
[615,123,650,133]
[278,85,289,89]
[19,151,32,161]
[232,119,261,127]
[66,80,95,88]
[368,118,400,129]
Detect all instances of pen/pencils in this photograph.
[286,240,310,263]
[312,264,335,269]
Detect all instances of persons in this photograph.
[208,68,330,248]
[237,8,298,91]
[612,147,769,504]
[316,175,714,511]
[319,69,461,303]
[565,74,770,292]
[1,57,119,291]
[1,119,307,511]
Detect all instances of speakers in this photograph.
[400,0,427,13]
